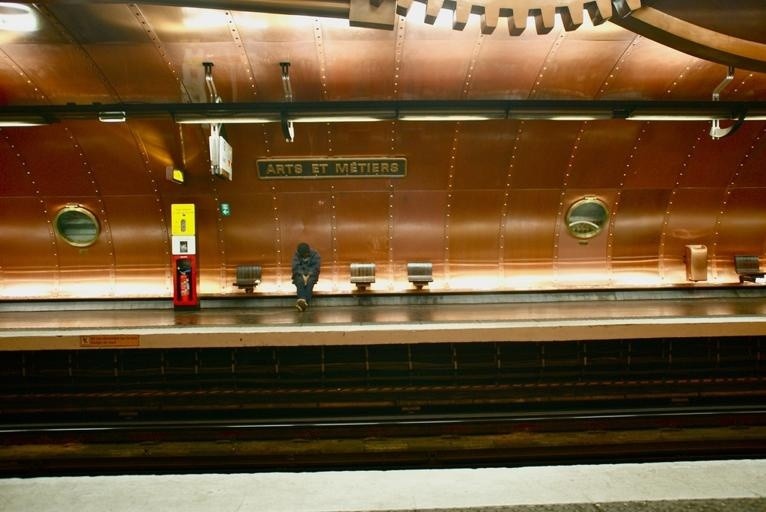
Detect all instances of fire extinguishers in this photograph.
[179,269,190,302]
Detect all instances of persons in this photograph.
[291,242,321,313]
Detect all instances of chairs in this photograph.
[350,263,376,295]
[734,255,766,284]
[232,265,262,296]
[406,263,434,294]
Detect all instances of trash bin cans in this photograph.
[685,244,708,282]
